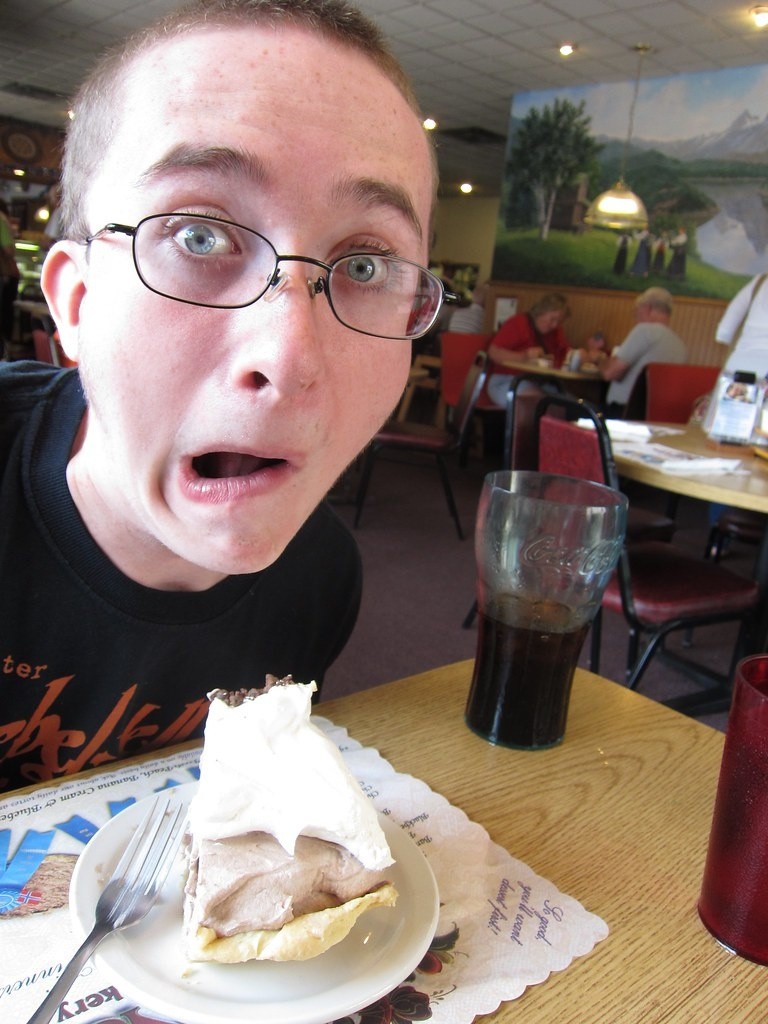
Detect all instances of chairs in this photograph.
[461,372,570,632]
[534,392,761,719]
[31,329,72,368]
[351,349,490,544]
[437,329,508,461]
[641,361,721,425]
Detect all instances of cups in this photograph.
[696,653,768,967]
[463,468,628,752]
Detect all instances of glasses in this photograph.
[81,213,472,341]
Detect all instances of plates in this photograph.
[68,780,441,1024]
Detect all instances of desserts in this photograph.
[179,675,397,964]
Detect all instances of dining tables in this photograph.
[0,657,768,1024]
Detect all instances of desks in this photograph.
[13,300,62,367]
[500,358,624,418]
[571,420,768,719]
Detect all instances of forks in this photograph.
[25,797,191,1024]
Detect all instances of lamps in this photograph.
[582,41,657,231]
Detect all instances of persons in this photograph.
[0,0,458,792]
[435,274,490,335]
[591,286,688,420]
[613,228,688,280]
[0,199,19,359]
[724,383,749,401]
[489,290,587,460]
[692,272,768,444]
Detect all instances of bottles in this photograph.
[718,370,760,446]
